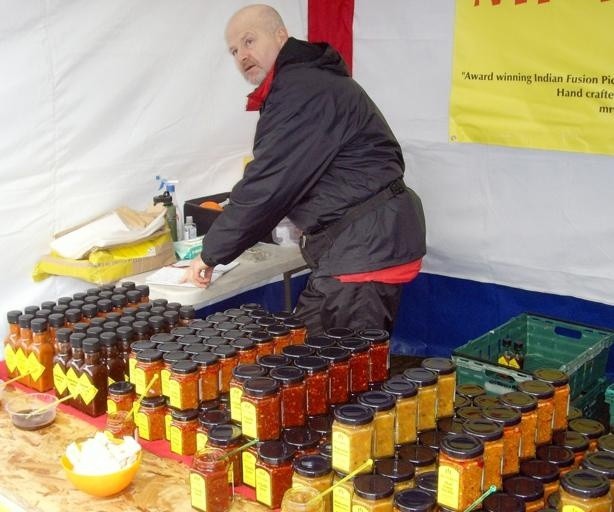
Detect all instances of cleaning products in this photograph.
[156,173,184,242]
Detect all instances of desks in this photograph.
[116,234,310,314]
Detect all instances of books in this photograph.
[147,258,240,288]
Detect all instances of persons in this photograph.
[179,5,426,336]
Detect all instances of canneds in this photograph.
[464,418,504,492]
[162,351,189,397]
[381,380,418,444]
[457,407,482,420]
[156,342,182,352]
[207,312,228,324]
[256,316,279,327]
[421,358,456,417]
[221,330,244,343]
[598,434,614,450]
[190,320,212,330]
[192,352,219,401]
[203,337,227,347]
[355,329,390,382]
[396,445,436,473]
[195,328,220,339]
[184,344,208,354]
[418,428,449,451]
[553,431,588,464]
[258,354,291,369]
[532,368,571,431]
[190,447,234,512]
[352,475,395,512]
[396,488,435,512]
[521,459,560,502]
[483,492,527,512]
[150,333,175,343]
[293,356,329,415]
[316,347,350,404]
[282,427,320,457]
[282,485,325,512]
[457,383,484,398]
[456,394,471,409]
[536,445,579,476]
[243,441,262,490]
[241,376,282,440]
[171,408,199,457]
[256,441,292,508]
[518,380,554,444]
[293,454,332,512]
[333,474,352,512]
[270,365,308,428]
[332,404,374,476]
[200,399,220,412]
[304,335,335,348]
[437,416,464,434]
[403,368,436,431]
[284,318,307,345]
[128,340,156,384]
[282,345,314,357]
[170,360,199,410]
[230,365,268,423]
[228,338,258,365]
[249,309,268,318]
[324,327,354,339]
[357,391,395,457]
[135,395,167,441]
[224,308,245,318]
[134,349,161,398]
[504,476,545,512]
[240,325,264,337]
[266,325,291,353]
[176,335,202,345]
[200,409,229,427]
[569,406,582,420]
[437,434,484,511]
[211,345,238,393]
[583,451,613,494]
[500,391,538,459]
[374,458,415,492]
[415,471,439,493]
[482,407,522,476]
[309,412,332,440]
[336,336,371,392]
[171,327,195,338]
[240,303,262,315]
[559,467,613,512]
[205,424,243,487]
[474,395,499,408]
[214,321,239,331]
[568,417,605,437]
[108,382,135,434]
[231,315,255,327]
[249,332,274,357]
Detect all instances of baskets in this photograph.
[573,379,607,421]
[450,313,614,399]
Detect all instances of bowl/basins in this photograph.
[60,436,140,498]
[6,392,58,430]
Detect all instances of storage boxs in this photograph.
[183,191,233,234]
[450,313,614,421]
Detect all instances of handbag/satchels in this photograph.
[299,228,330,269]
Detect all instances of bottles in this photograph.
[3,310,21,380]
[117,325,137,377]
[28,317,53,392]
[52,328,72,405]
[23,281,195,331]
[152,192,176,244]
[82,337,107,416]
[100,332,121,381]
[17,314,34,386]
[182,216,197,241]
[64,332,87,410]
